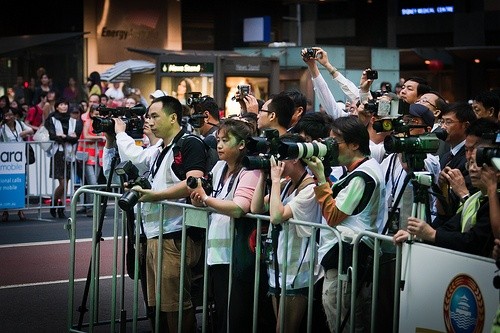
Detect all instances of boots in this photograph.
[50,199,58,218]
[58,201,67,219]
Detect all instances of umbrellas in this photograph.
[99,58,155,86]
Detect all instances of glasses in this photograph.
[413,98,438,110]
[256,107,279,118]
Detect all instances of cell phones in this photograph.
[366,70,378,79]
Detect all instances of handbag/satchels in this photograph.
[126,234,147,279]
[33,115,56,151]
[18,119,36,165]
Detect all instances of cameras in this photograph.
[186,91,203,107]
[307,48,318,58]
[115,160,152,211]
[373,98,410,132]
[383,131,440,154]
[363,99,380,116]
[412,171,434,188]
[476,146,500,172]
[186,171,213,197]
[240,129,340,173]
[237,84,249,99]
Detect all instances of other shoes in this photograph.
[86,209,93,217]
[75,208,87,214]
[18,212,25,221]
[0,213,9,221]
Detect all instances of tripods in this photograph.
[338,166,432,333]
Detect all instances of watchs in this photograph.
[203,196,209,207]
[461,193,471,203]
[331,67,337,75]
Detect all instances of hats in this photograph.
[491,156,500,170]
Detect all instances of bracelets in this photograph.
[318,183,321,186]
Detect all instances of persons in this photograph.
[0,47,500,333]
